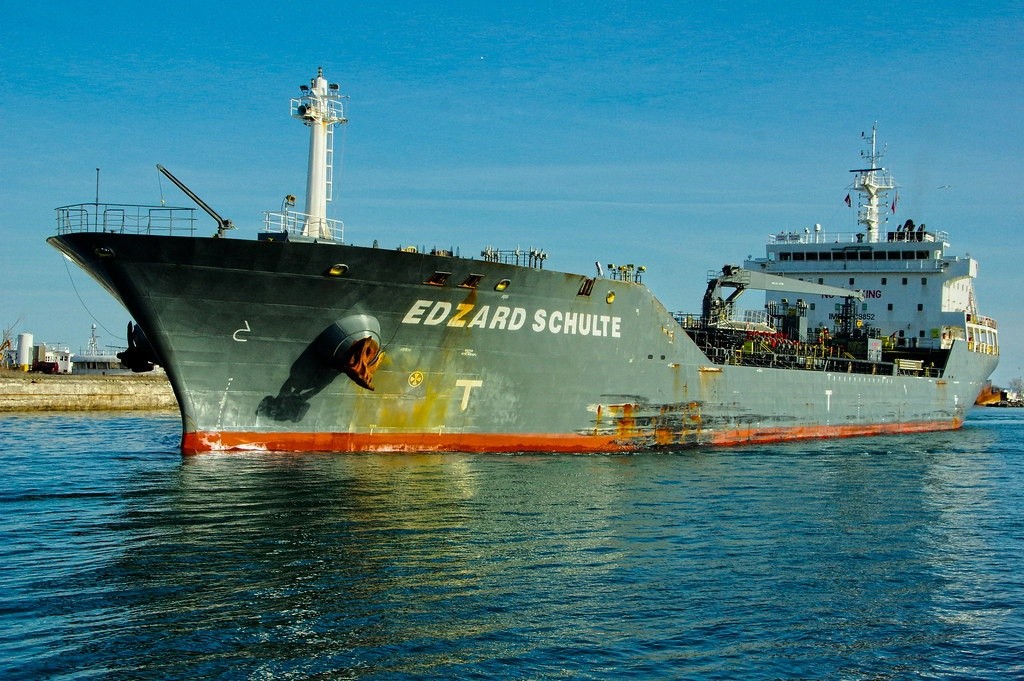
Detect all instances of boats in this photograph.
[44,68,1000,452]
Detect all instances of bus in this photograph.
[70,354,132,375]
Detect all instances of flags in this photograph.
[892,202,896,214]
[845,193,851,207]
